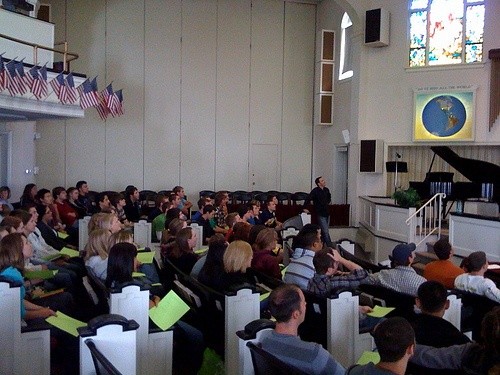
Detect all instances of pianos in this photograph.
[408,146,500,225]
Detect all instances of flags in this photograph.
[5,60,19,96]
[17,61,28,95]
[77,78,100,110]
[39,65,48,97]
[96,84,124,120]
[25,67,42,100]
[50,73,77,105]
[0,55,6,91]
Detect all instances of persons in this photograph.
[411,308,500,375]
[0,181,382,375]
[408,280,471,347]
[259,284,346,375]
[366,242,427,296]
[303,177,332,247]
[345,317,416,375]
[423,239,465,287]
[454,251,500,303]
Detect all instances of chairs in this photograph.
[136,188,310,206]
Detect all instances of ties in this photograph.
[33,230,40,238]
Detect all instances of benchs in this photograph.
[0,216,500,375]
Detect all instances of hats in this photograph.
[393,243,417,259]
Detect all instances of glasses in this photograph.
[47,209,51,215]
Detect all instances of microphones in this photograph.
[396,151,402,158]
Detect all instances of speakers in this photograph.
[365,8,390,46]
[360,139,383,173]
